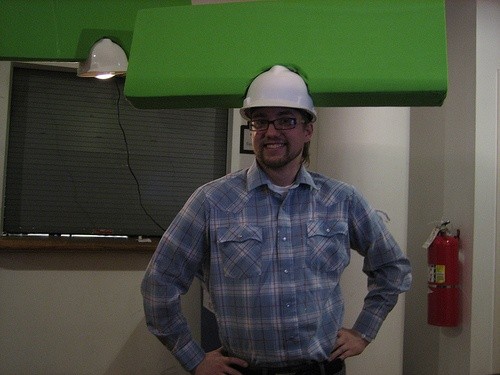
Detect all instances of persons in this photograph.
[140,65,413,375]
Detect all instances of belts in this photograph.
[231,359,342,375]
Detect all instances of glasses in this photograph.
[247,117,306,132]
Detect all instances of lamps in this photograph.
[77,39,129,80]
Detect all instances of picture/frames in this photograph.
[240,125,255,155]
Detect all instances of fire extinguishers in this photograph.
[422,220,461,328]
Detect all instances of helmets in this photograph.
[240,65,317,123]
